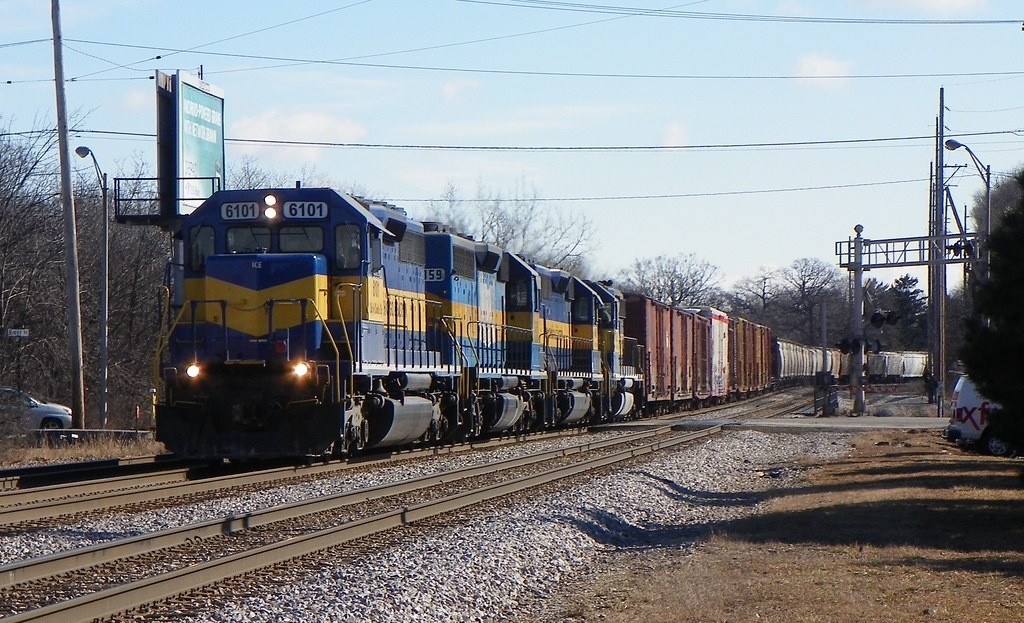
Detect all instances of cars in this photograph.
[0,385,73,448]
[940,374,1024,459]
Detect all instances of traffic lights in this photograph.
[884,310,902,326]
[870,312,885,328]
[846,338,860,354]
[834,338,850,354]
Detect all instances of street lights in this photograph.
[944,138,993,330]
[75,144,111,437]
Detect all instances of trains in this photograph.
[153,179,932,479]
